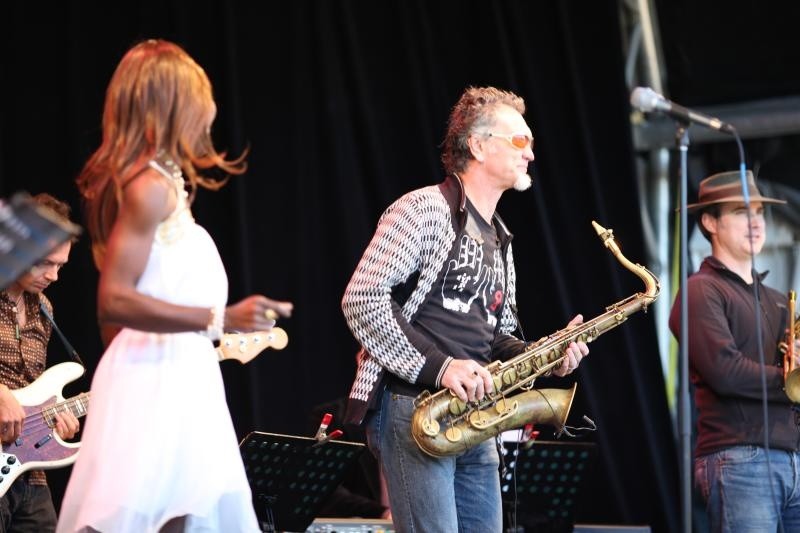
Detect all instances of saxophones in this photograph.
[414,221,659,459]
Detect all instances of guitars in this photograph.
[0,326,289,498]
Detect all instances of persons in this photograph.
[0,195,79,533]
[55,38,293,532]
[669,170,800,533]
[342,88,591,533]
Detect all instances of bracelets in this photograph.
[208,306,224,339]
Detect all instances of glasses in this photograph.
[473,131,535,150]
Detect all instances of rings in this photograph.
[265,310,275,319]
[569,367,577,370]
[4,428,7,431]
[472,371,478,377]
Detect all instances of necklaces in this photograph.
[158,149,183,178]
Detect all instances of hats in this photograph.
[674,170,789,213]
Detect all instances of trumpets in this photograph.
[778,290,800,404]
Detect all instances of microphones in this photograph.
[564,416,596,431]
[630,87,737,134]
